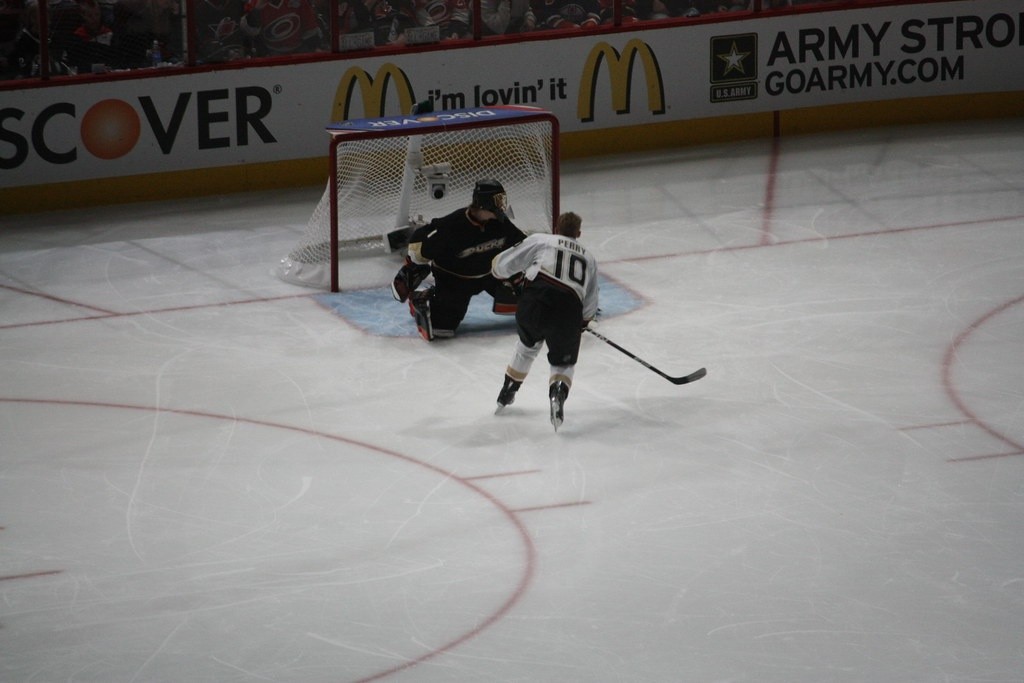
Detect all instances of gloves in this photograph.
[581,321,589,333]
[503,272,524,295]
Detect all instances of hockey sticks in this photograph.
[585,326,707,385]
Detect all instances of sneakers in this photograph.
[495,380,520,415]
[551,380,564,431]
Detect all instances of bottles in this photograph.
[151,40,161,70]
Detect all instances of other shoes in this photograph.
[493,300,519,315]
[409,287,455,341]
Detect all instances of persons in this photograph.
[392,179,528,341]
[1,0,794,83]
[491,211,600,431]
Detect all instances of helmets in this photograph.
[472,180,507,221]
